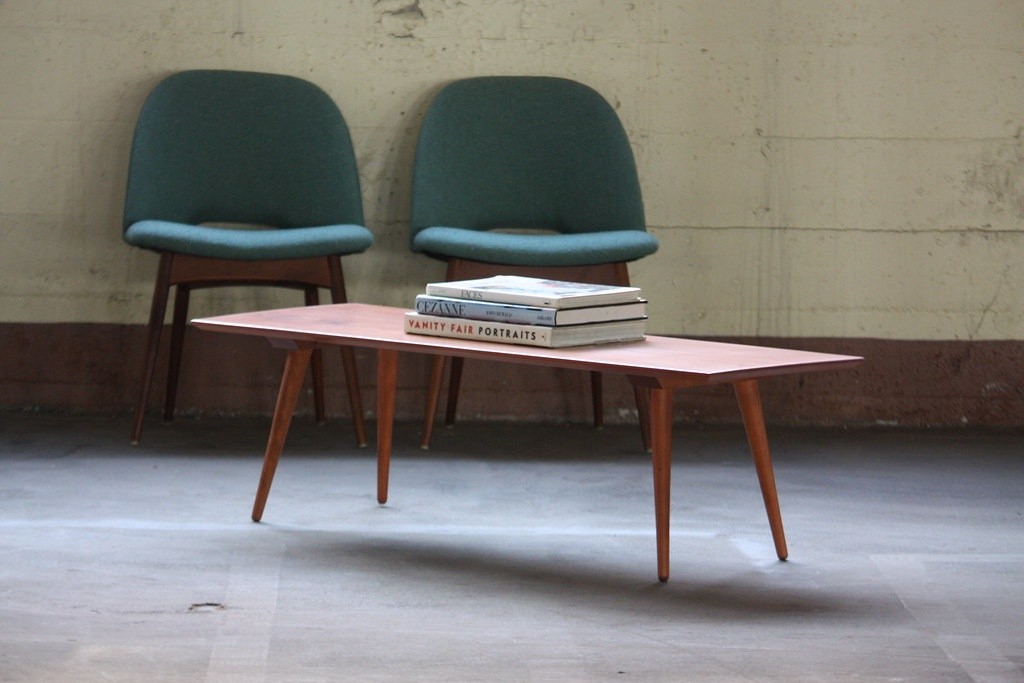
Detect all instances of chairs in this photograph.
[404,74,667,454]
[119,68,370,446]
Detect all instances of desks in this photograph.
[179,300,867,580]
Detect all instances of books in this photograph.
[415,294,648,326]
[426,275,642,308]
[404,312,646,349]
